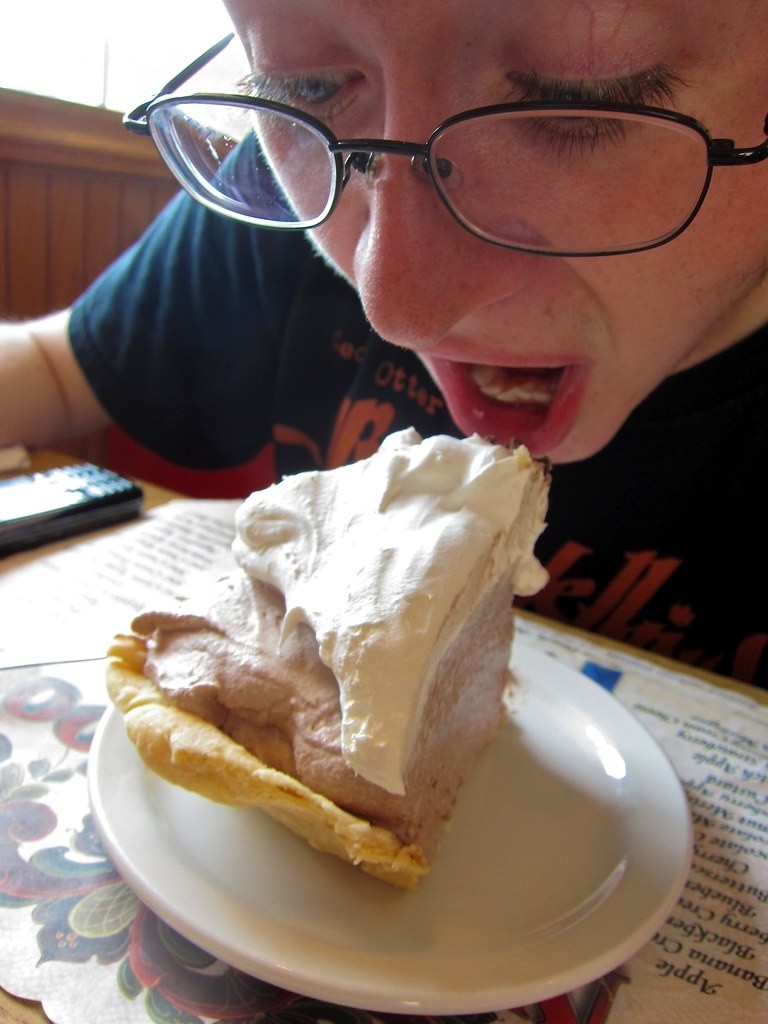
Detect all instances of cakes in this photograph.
[105,426,551,889]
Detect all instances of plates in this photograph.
[87,642,693,1012]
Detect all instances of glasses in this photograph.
[121,33,768,256]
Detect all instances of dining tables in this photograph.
[0,447,768,1024]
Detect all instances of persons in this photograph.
[0,0,768,697]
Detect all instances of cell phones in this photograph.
[0,454,145,552]
